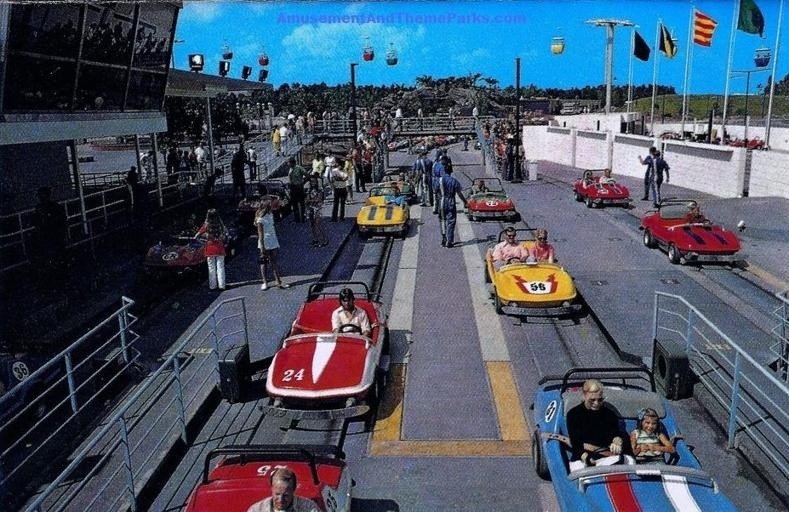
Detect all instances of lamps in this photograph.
[188,54,205,73]
[218,61,230,77]
[241,65,252,80]
[259,70,268,82]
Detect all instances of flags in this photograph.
[658,24,678,60]
[736,0,765,38]
[691,8,719,48]
[632,30,651,61]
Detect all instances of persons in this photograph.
[640,150,670,203]
[246,467,323,512]
[566,378,637,473]
[528,228,555,264]
[330,288,372,338]
[629,407,676,465]
[685,206,705,221]
[637,146,656,201]
[599,168,615,188]
[24,103,530,292]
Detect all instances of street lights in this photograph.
[349,61,360,144]
[583,18,638,116]
[511,55,523,183]
[234,101,272,133]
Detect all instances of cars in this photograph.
[355,186,411,240]
[528,364,736,512]
[372,175,415,204]
[639,197,747,266]
[145,213,241,272]
[387,135,474,155]
[663,129,767,150]
[182,443,354,512]
[484,226,584,319]
[259,278,389,423]
[572,169,632,208]
[463,176,516,223]
[237,177,294,222]
